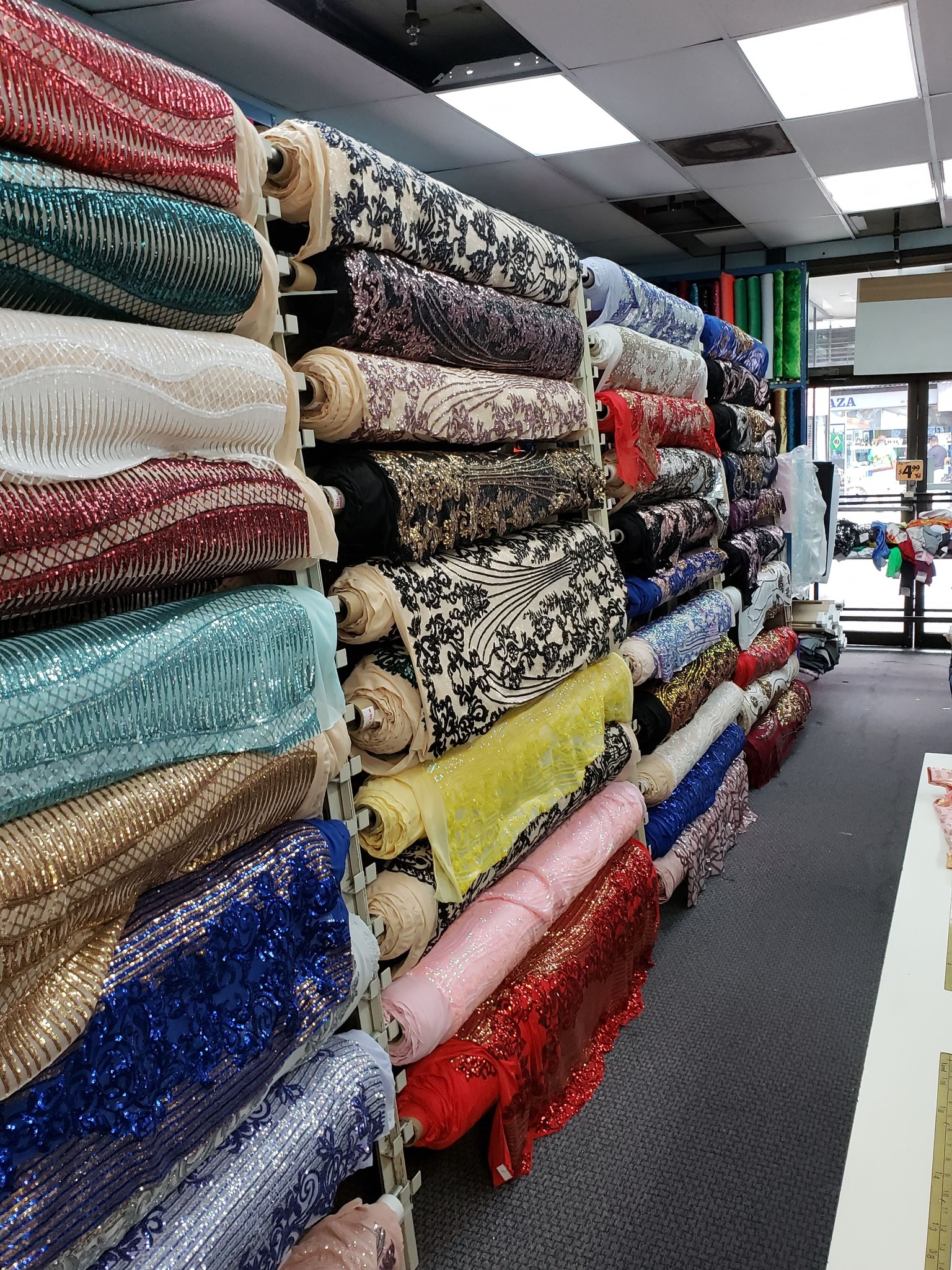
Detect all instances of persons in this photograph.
[928,435,947,483]
[868,435,897,464]
